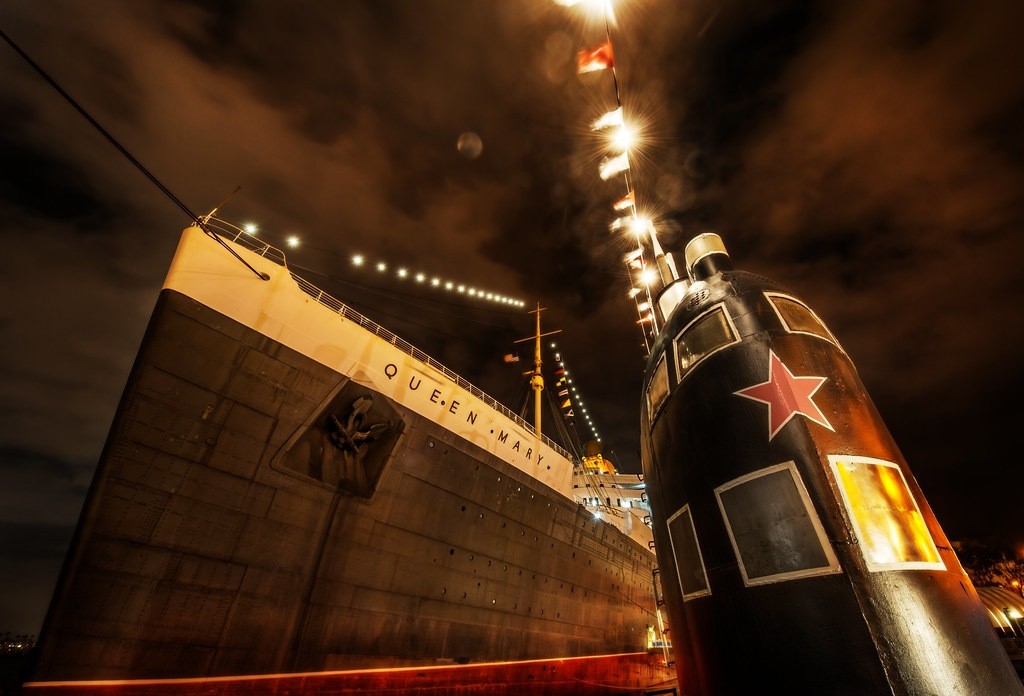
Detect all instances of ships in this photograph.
[19,184,670,689]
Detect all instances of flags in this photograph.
[594,106,623,129]
[612,190,635,212]
[503,352,519,362]
[554,369,564,375]
[575,40,616,74]
[555,377,567,387]
[598,151,630,181]
[558,399,571,408]
[558,389,569,396]
[564,409,575,418]
